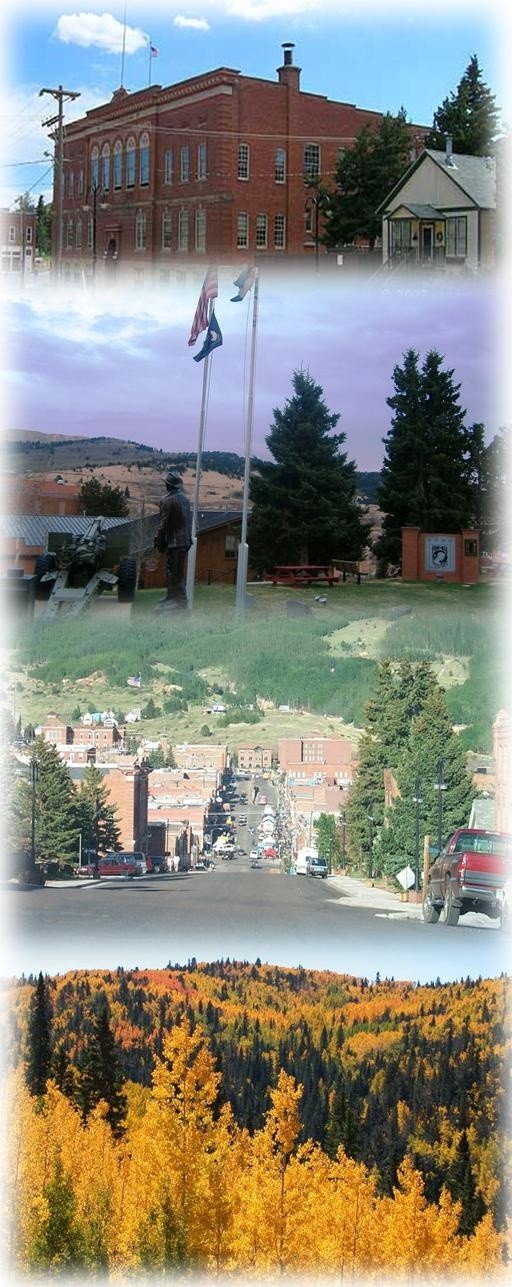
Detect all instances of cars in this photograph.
[146,767,281,873]
[76,851,167,877]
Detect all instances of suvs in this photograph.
[307,858,327,879]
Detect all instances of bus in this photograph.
[296,847,319,875]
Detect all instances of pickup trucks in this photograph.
[423,828,508,927]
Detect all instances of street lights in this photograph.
[82,182,109,264]
[368,802,374,879]
[28,759,40,869]
[310,810,315,847]
[434,758,448,849]
[413,774,423,889]
[342,811,347,868]
[304,193,332,270]
[327,830,334,874]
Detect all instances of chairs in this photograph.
[495,843,507,854]
[462,845,474,852]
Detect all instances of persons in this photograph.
[152,469,195,610]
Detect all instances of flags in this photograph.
[230,263,258,302]
[150,46,159,58]
[193,310,224,362]
[187,260,217,347]
